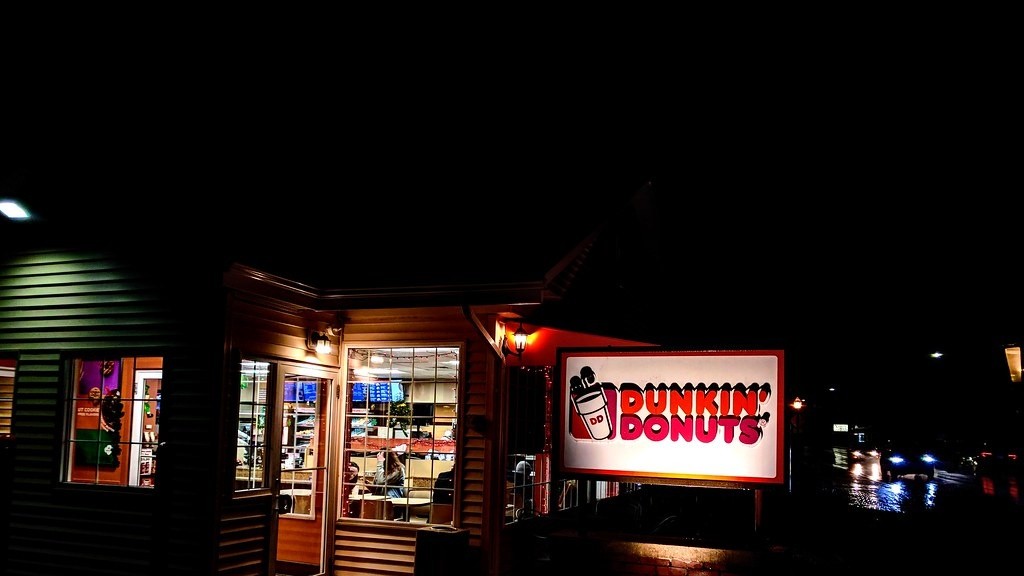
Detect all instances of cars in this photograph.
[879,448,937,482]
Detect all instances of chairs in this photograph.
[359,499,394,521]
[429,504,453,526]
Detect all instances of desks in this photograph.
[348,496,434,522]
[280,489,311,515]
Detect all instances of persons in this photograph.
[347,462,359,496]
[362,448,405,519]
[516,461,531,480]
[427,464,455,523]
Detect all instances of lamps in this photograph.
[311,332,331,353]
[501,321,528,359]
